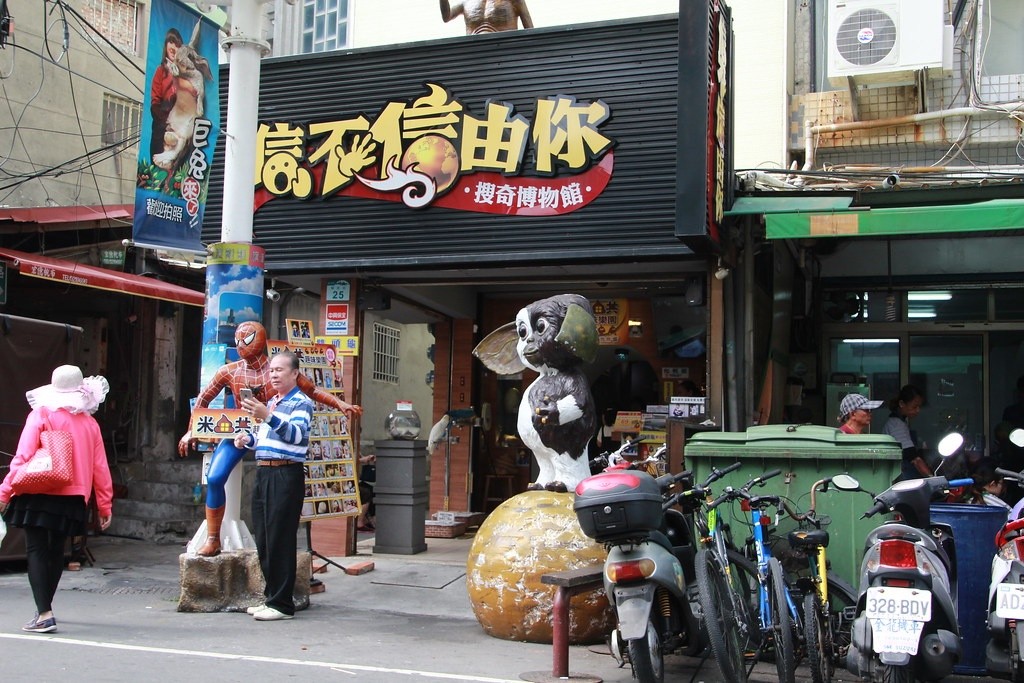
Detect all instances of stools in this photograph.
[482,475,514,512]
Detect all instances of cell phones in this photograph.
[239,388,252,402]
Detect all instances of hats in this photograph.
[839,393,884,416]
[25,364,111,416]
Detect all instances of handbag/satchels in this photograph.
[10,405,73,496]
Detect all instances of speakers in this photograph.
[357,286,391,310]
[684,273,705,308]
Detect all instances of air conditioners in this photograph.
[827,1,946,90]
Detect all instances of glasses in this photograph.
[857,408,870,413]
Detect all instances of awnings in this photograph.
[0,248,205,306]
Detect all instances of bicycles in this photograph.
[661,460,763,683]
[704,469,806,683]
[588,435,666,479]
[778,472,859,683]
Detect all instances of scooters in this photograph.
[982,429,1024,683]
[830,430,974,683]
[573,470,706,683]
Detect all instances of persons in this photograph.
[943,406,1024,513]
[882,384,933,479]
[0,364,113,631]
[179,319,364,557]
[151,26,183,167]
[837,394,885,434]
[596,413,618,453]
[234,351,313,622]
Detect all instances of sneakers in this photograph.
[254,607,295,621]
[246,603,269,615]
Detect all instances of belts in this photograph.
[256,459,295,466]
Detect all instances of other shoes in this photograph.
[22,616,57,632]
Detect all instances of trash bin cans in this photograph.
[683,421,903,615]
[929,504,1009,677]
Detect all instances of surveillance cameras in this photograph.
[266,289,280,302]
[715,268,730,280]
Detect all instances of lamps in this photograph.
[909,310,941,319]
[685,278,708,306]
[907,290,954,301]
[358,286,391,312]
